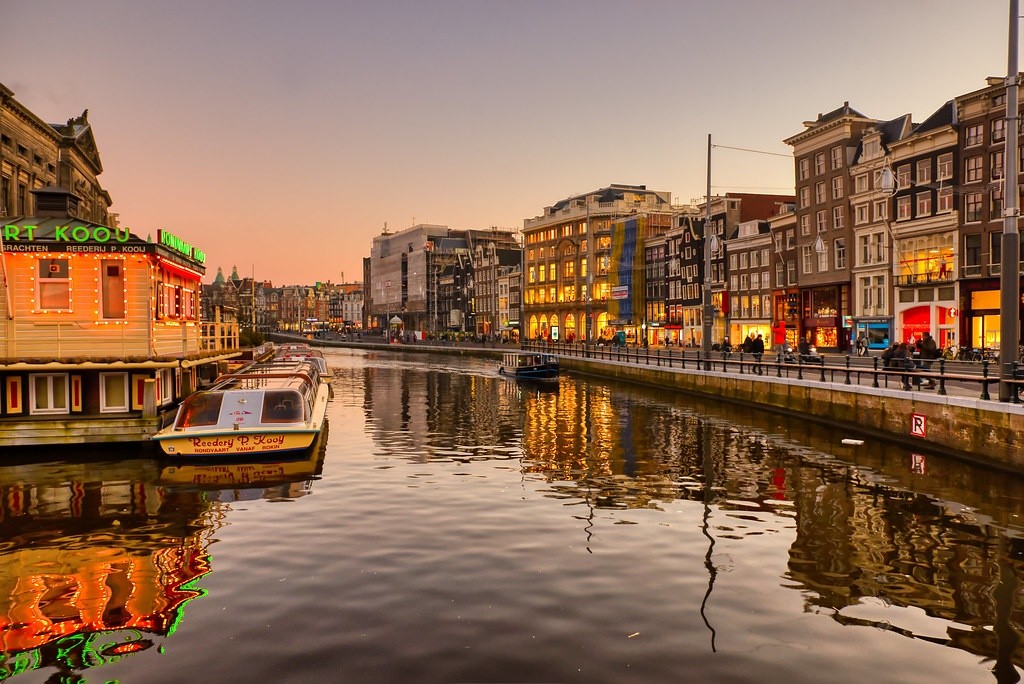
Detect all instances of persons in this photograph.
[662,335,672,348]
[405,330,623,353]
[856,335,872,357]
[880,331,940,390]
[720,331,765,375]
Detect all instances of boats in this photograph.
[149,360,329,457]
[152,418,330,491]
[262,341,335,386]
[495,351,561,379]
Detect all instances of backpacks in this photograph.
[859,340,863,347]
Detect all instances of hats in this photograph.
[921,332,931,338]
[801,337,805,340]
[724,336,728,340]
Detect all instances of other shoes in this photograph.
[866,356,869,357]
[920,380,929,386]
[903,384,912,389]
[858,354,863,356]
[751,369,757,373]
[758,370,763,375]
[863,354,865,356]
[924,380,937,390]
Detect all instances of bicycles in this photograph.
[941,342,1024,368]
